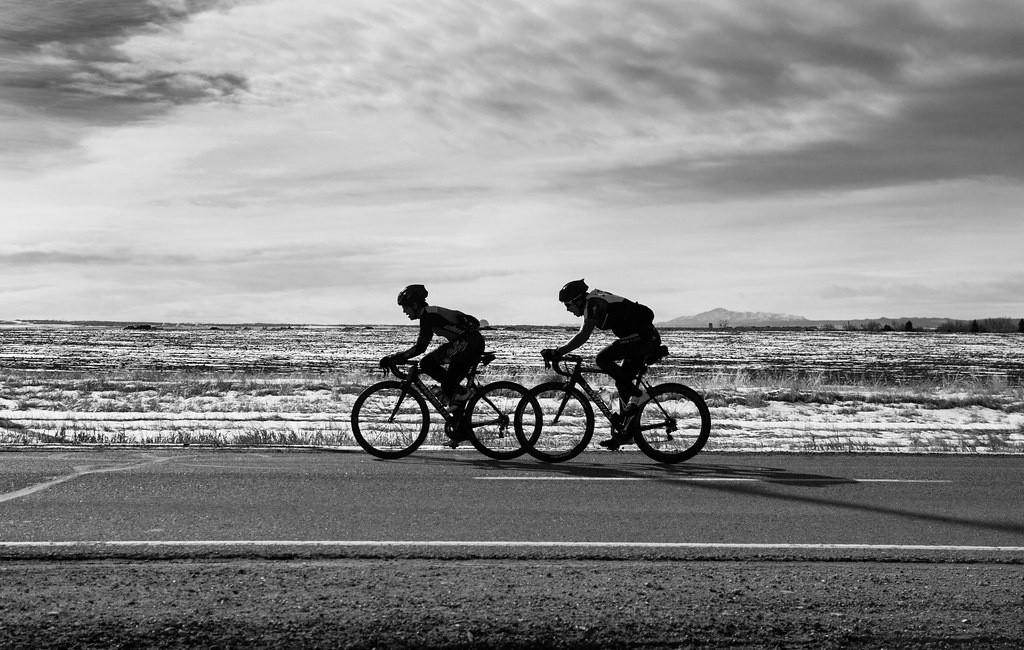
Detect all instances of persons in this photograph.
[540,279,661,447]
[380,285,485,445]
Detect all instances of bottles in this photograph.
[429,384,449,406]
[597,387,619,415]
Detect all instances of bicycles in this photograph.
[514,344,712,463]
[350,351,543,461]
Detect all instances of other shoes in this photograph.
[600,435,633,449]
[446,387,474,414]
[620,391,651,417]
[442,437,456,445]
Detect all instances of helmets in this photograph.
[396,284,428,306]
[558,278,589,303]
